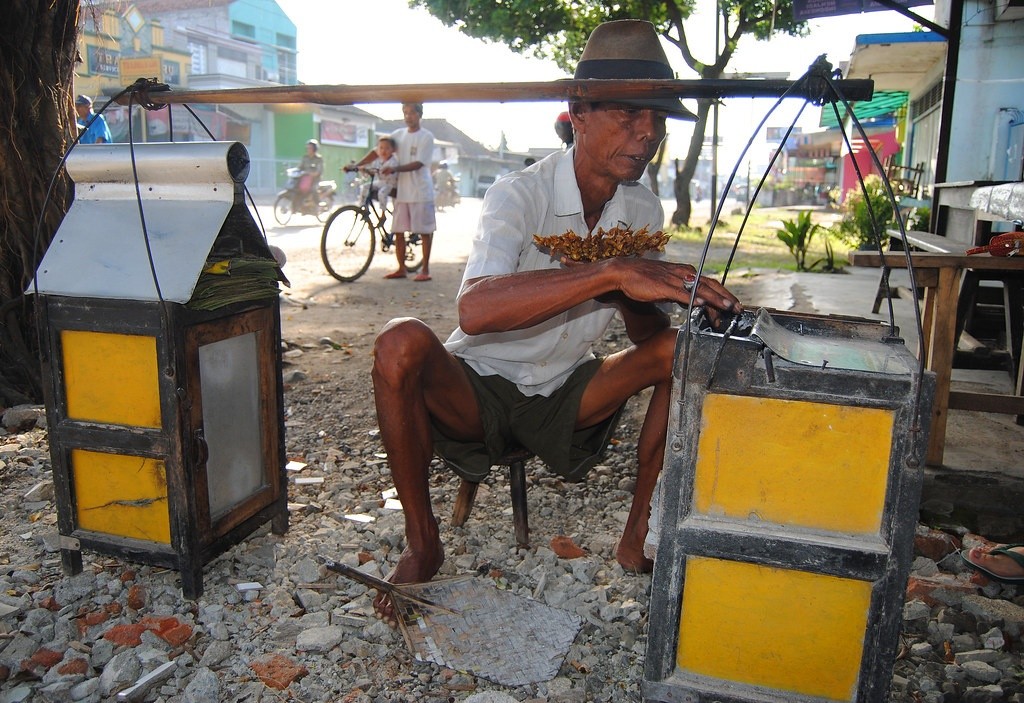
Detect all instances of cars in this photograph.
[687,179,750,203]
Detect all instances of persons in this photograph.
[429,160,455,213]
[291,138,323,215]
[74,94,113,144]
[554,111,575,150]
[357,137,399,228]
[371,22,751,629]
[343,100,438,281]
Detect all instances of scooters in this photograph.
[433,171,463,212]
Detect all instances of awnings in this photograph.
[788,91,907,159]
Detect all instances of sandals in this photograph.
[961,543,1023,580]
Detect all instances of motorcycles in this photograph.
[274,162,338,226]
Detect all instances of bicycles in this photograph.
[321,164,424,282]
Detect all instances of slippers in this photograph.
[385,273,407,279]
[414,274,432,282]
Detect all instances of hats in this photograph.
[75,94,92,104]
[560,19,700,122]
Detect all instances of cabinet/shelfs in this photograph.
[32,288,292,604]
[633,303,938,703]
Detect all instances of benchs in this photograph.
[845,226,1024,471]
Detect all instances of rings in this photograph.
[683,273,695,292]
